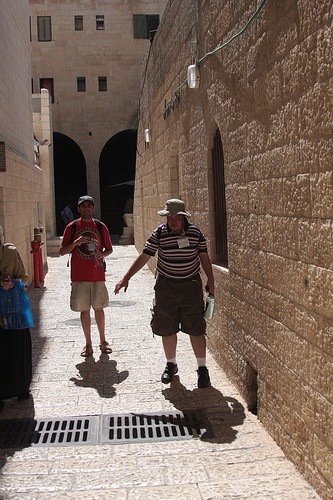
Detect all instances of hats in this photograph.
[78,195,94,206]
[158,199,191,218]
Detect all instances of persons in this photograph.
[114,199,215,388]
[59,196,113,357]
[0,232,33,401]
[61,201,75,227]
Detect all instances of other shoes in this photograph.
[0,395,18,402]
[196,369,211,388]
[161,362,178,384]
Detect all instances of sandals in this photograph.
[100,343,112,353]
[81,346,93,356]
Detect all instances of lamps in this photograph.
[144,128,151,143]
[33,136,49,145]
[187,64,200,89]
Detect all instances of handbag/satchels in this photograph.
[0,279,35,330]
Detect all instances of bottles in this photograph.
[205,294,215,319]
[92,249,104,268]
